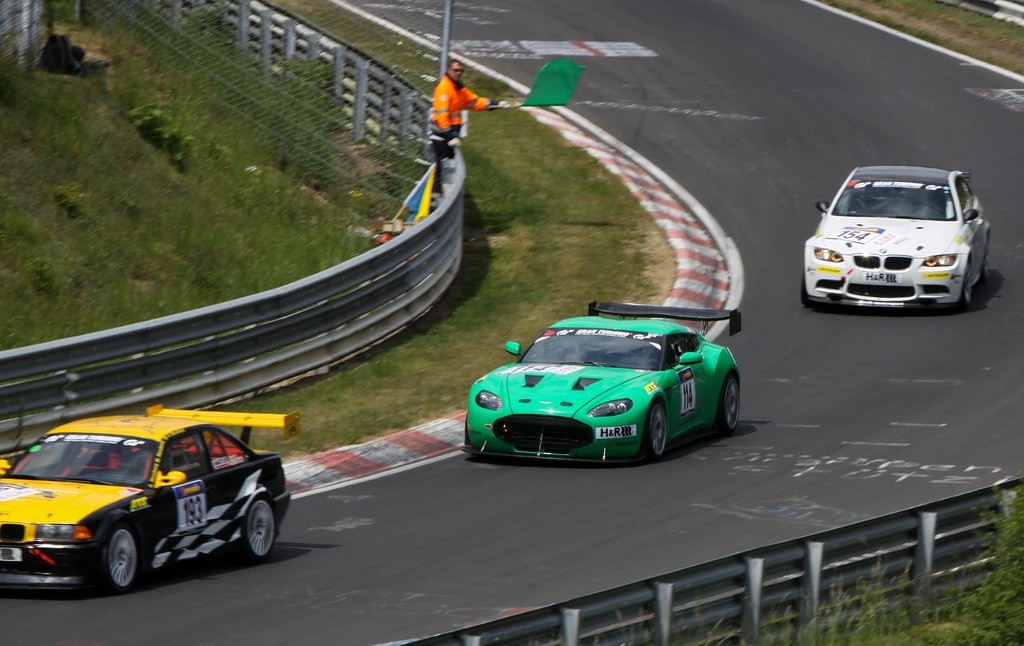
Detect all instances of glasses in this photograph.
[451,68,464,74]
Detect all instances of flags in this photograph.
[516,58,587,106]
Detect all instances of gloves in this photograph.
[498,101,512,110]
[447,138,462,149]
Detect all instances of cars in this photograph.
[800,165,991,313]
[0,404,303,596]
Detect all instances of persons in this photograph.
[428,58,514,199]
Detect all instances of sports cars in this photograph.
[459,300,742,467]
